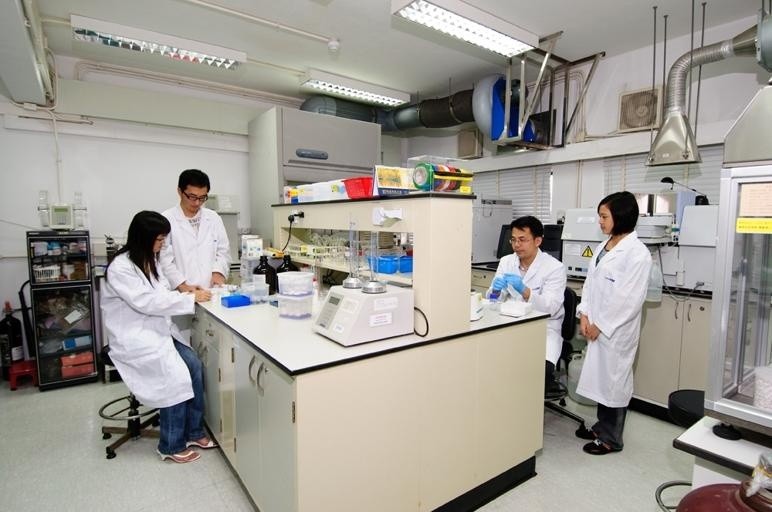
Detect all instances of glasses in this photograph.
[181,188,208,204]
[508,237,535,246]
[154,235,168,244]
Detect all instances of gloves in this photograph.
[491,275,508,293]
[503,271,525,294]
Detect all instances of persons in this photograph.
[485,215,567,398]
[156,169,231,346]
[101,210,219,463]
[575,190,652,456]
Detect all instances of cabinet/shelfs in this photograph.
[271,192,477,335]
[191,327,234,440]
[471,285,487,298]
[567,281,584,295]
[631,290,712,408]
[471,262,501,288]
[205,323,222,351]
[192,315,209,335]
[235,344,297,512]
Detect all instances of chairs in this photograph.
[545,286,586,430]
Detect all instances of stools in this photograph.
[10,360,39,390]
[655,389,704,512]
[99,344,161,459]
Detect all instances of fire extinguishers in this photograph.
[0,302,32,381]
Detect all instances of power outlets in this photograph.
[291,209,299,225]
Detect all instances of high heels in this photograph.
[185,439,221,449]
[154,445,202,464]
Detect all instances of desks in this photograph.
[673,417,771,492]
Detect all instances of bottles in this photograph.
[254,255,278,295]
[275,253,300,294]
[36,189,50,227]
[70,190,90,231]
[644,256,664,304]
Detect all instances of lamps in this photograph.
[660,176,708,205]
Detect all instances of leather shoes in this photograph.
[582,440,622,456]
[575,427,596,440]
